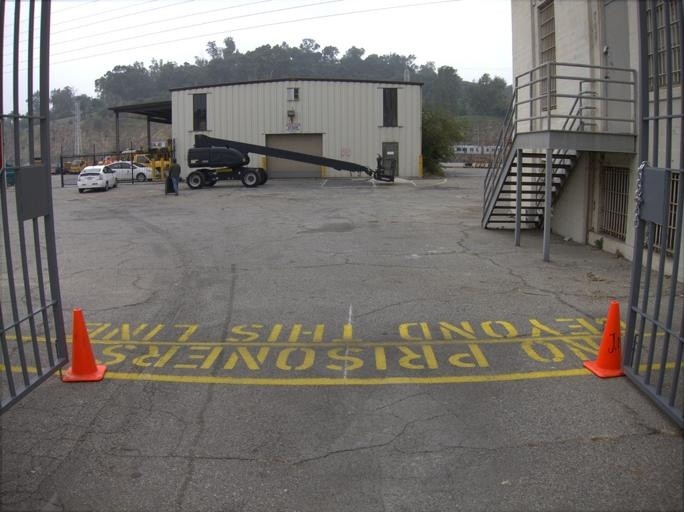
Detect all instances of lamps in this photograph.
[288,110,295,122]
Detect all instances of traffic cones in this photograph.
[582,299,626,378]
[62,308,107,381]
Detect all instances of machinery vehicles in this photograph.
[187,134,397,190]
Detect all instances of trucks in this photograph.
[70,160,87,174]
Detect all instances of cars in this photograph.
[108,162,160,181]
[77,167,117,192]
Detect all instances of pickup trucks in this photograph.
[106,154,169,169]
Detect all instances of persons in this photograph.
[98,156,113,165]
[169,158,181,196]
